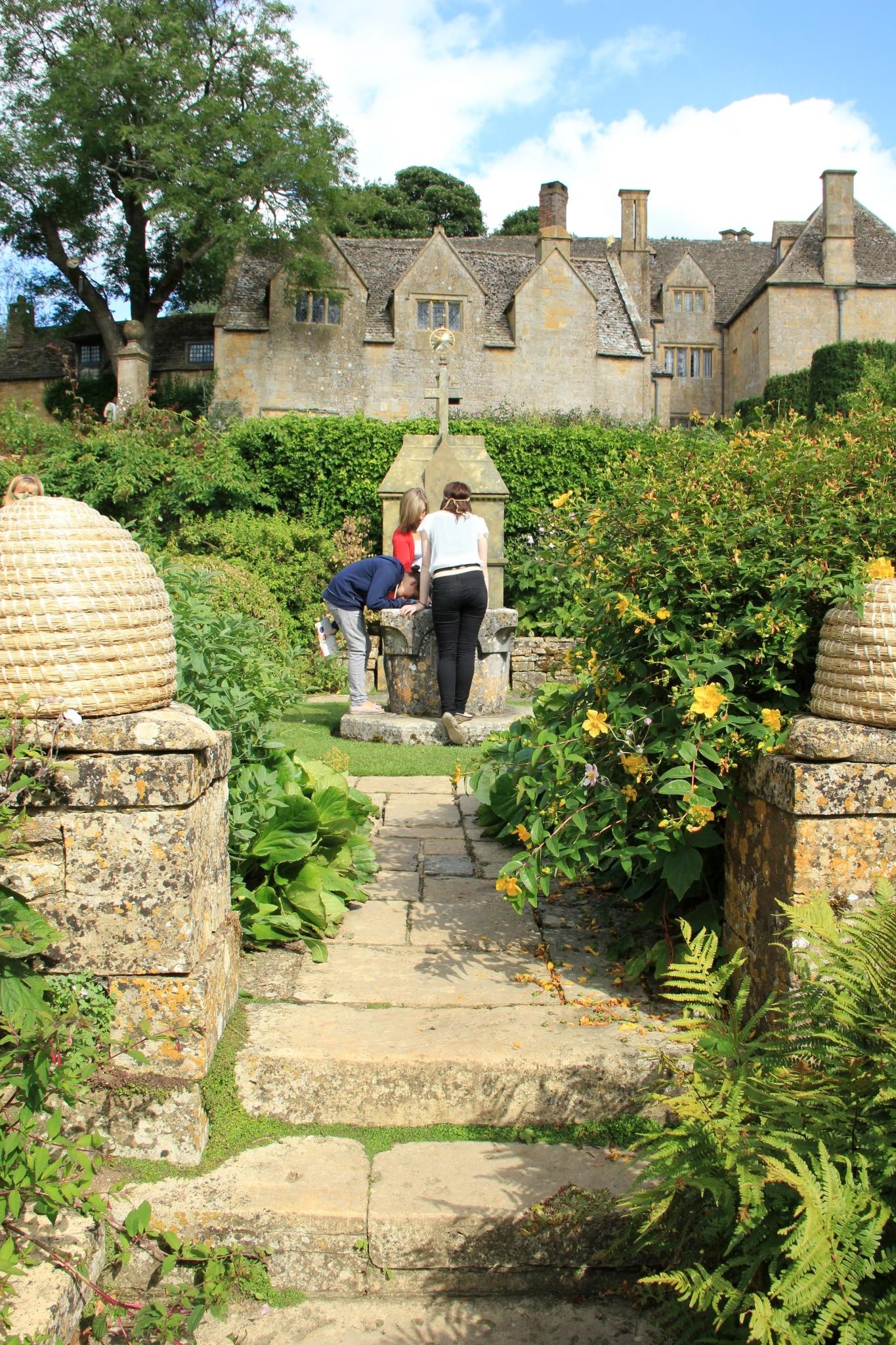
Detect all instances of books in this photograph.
[315,618,338,657]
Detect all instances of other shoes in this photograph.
[443,712,465,745]
[349,704,386,714]
[454,712,473,725]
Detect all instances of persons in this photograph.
[103,396,118,423]
[3,473,43,505]
[400,481,490,744]
[389,488,429,602]
[322,554,429,715]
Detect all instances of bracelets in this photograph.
[419,601,426,607]
